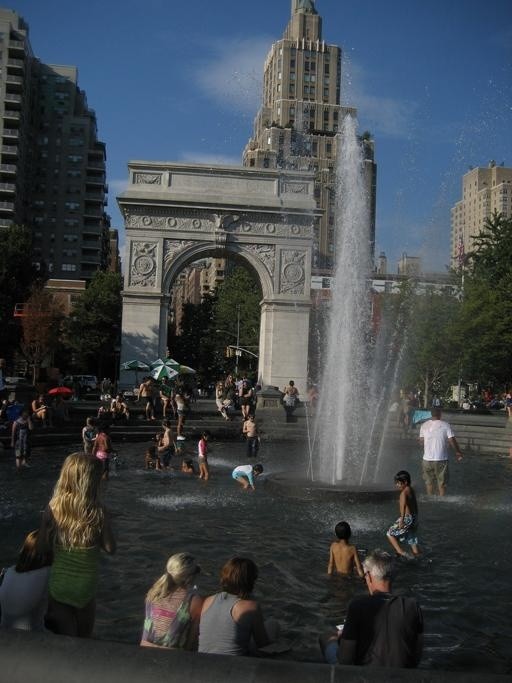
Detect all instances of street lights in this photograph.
[216,330,239,381]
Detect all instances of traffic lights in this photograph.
[223,346,230,358]
[229,347,235,358]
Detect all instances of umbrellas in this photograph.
[149,358,180,382]
[120,359,151,386]
[177,364,196,383]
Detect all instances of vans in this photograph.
[60,374,99,393]
